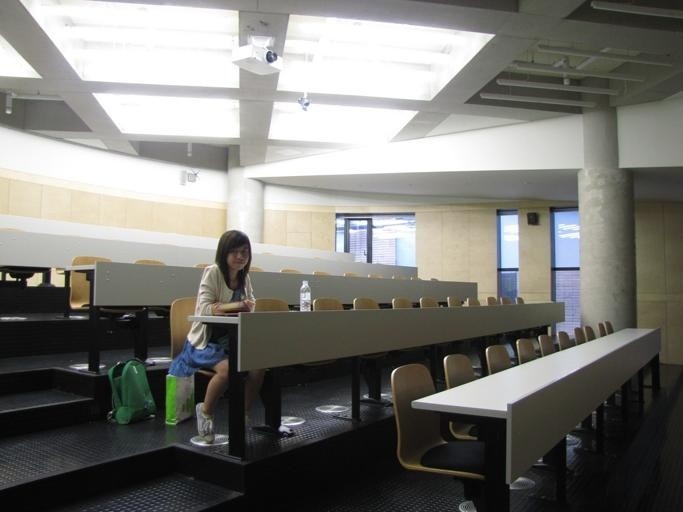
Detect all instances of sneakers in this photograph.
[195,402,216,444]
[244,413,254,434]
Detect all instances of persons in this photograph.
[168,230,265,444]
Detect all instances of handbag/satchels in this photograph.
[165,369,195,426]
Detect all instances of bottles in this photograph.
[301,280,312,311]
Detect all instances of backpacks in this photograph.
[105,357,158,425]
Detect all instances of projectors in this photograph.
[231,45,284,76]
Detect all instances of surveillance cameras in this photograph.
[298,97,311,108]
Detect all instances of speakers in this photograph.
[527,213,537,225]
